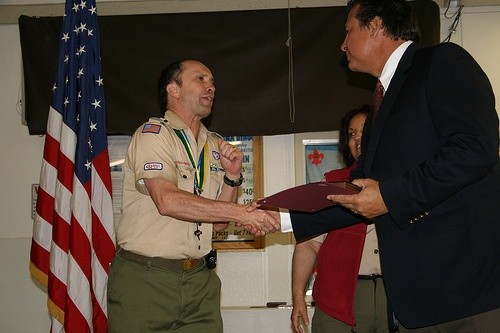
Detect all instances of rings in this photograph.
[233,145,237,151]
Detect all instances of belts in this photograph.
[115,238,205,271]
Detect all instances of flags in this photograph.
[29,0,116,333]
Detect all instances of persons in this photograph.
[289,105,390,333]
[106,60,281,333]
[236,0,500,333]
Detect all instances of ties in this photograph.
[369,79,384,131]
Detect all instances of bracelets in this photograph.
[224,173,243,187]
